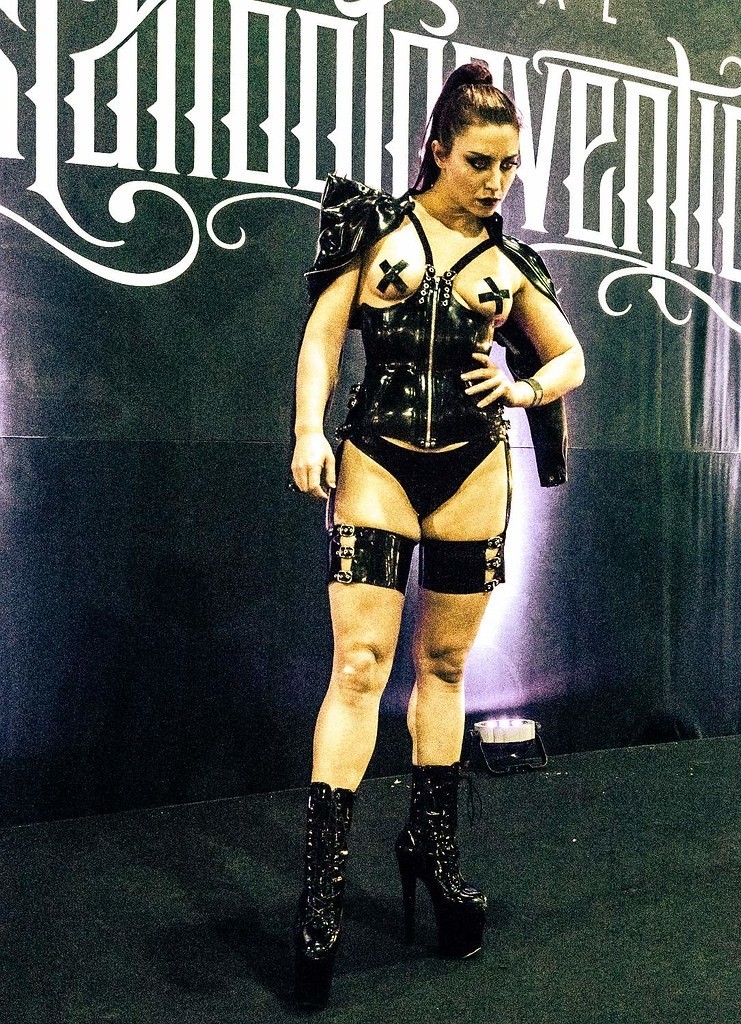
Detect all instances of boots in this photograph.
[292,783,357,961]
[396,761,488,959]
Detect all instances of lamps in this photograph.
[469,720,549,775]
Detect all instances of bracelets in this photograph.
[518,376,544,409]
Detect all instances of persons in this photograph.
[287,60,586,1014]
